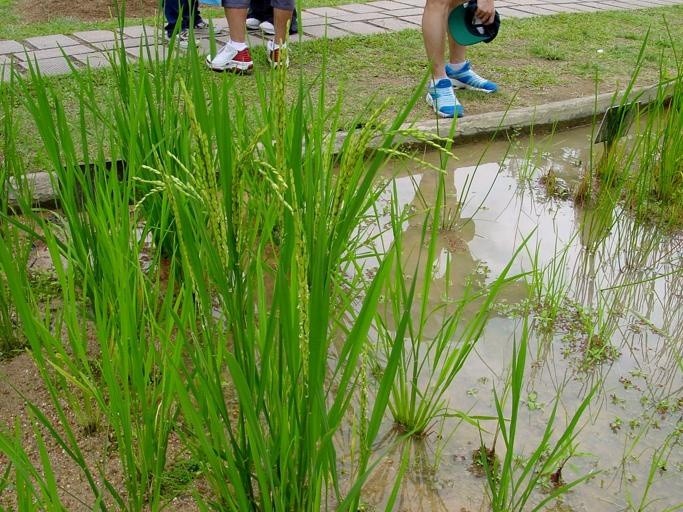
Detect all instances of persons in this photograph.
[421,1,501,120]
[245,0,300,35]
[378,172,482,346]
[162,1,224,50]
[204,0,297,77]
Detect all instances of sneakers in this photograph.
[246,18,274,35]
[165,22,220,49]
[267,39,289,68]
[206,42,253,70]
[426,60,498,117]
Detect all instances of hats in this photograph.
[447,2,500,46]
